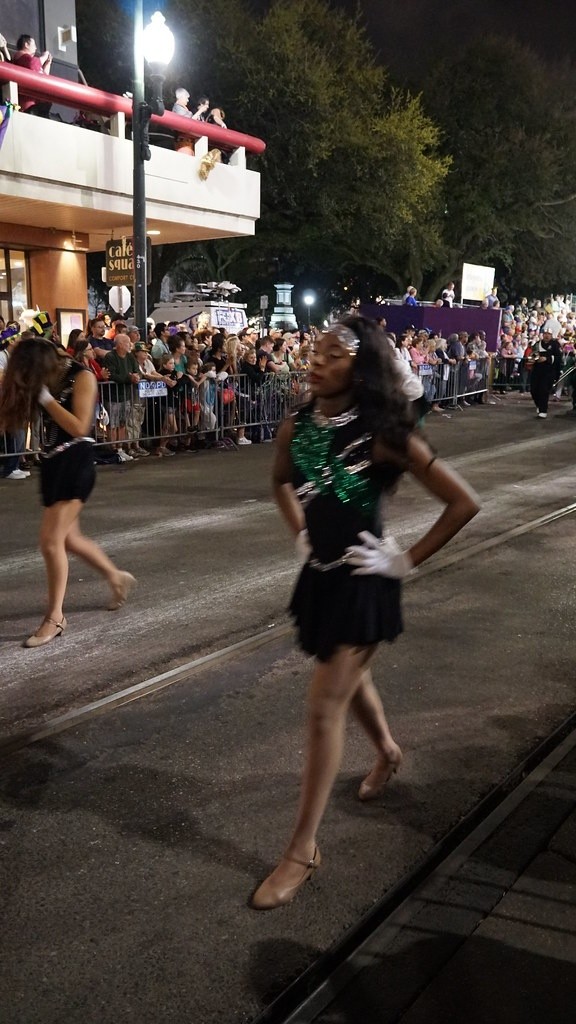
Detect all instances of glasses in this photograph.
[87,349,91,350]
[94,324,107,329]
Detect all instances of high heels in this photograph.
[26,617,67,647]
[251,844,323,910]
[107,571,136,611]
[359,747,403,798]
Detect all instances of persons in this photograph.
[0,337,140,647]
[0,304,320,479]
[526,328,563,418]
[12,34,54,119]
[172,88,229,164]
[250,315,482,909]
[378,282,576,416]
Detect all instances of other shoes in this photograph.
[463,401,471,406]
[13,470,31,476]
[7,473,26,479]
[433,405,445,411]
[537,408,539,412]
[538,413,548,418]
[186,445,198,452]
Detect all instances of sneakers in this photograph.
[136,448,150,456]
[128,449,138,457]
[237,437,252,445]
[157,448,175,456]
[119,449,133,461]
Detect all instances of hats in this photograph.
[134,341,149,351]
[246,328,260,335]
[542,328,552,334]
[128,325,140,332]
[459,331,470,338]
[269,328,283,337]
[1,326,22,344]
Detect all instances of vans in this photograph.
[148,280,249,337]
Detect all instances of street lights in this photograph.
[304,295,315,326]
[126,1,178,345]
